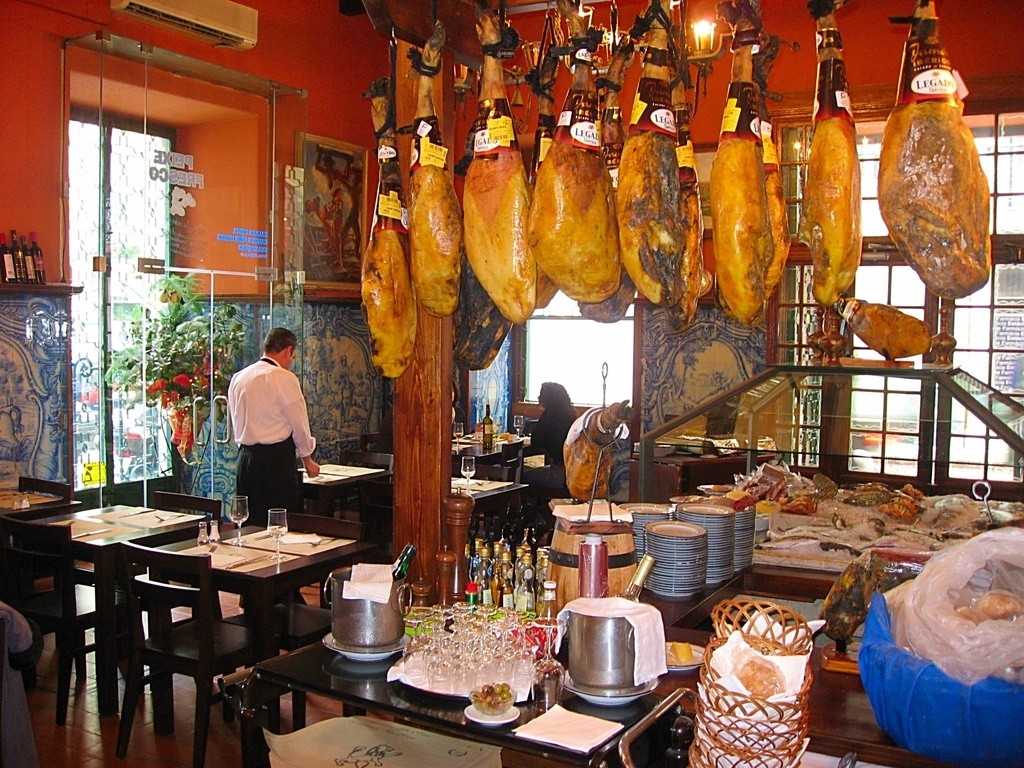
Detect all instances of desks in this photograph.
[630,447,779,501]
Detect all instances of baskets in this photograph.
[698,666,813,719]
[692,733,809,768]
[693,709,810,754]
[704,636,812,698]
[687,739,716,768]
[712,596,813,655]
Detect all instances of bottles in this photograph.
[9,230,29,285]
[457,514,561,620]
[208,520,220,549]
[391,544,418,581]
[609,552,657,607]
[18,236,36,287]
[482,404,493,449]
[196,522,211,549]
[29,231,47,286]
[0,232,16,284]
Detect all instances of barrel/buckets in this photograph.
[543,515,640,612]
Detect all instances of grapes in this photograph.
[472,682,511,714]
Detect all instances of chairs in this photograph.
[1,418,553,767]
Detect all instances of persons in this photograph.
[224,328,320,608]
[522,382,580,541]
[452,380,468,440]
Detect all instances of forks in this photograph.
[207,545,217,554]
[154,514,186,521]
[307,538,337,546]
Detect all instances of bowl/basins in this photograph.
[469,686,517,714]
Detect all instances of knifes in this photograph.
[223,554,268,570]
[117,509,156,519]
[71,529,113,538]
[254,534,274,540]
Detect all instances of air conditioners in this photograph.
[110,0,261,52]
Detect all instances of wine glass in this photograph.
[228,496,250,547]
[396,601,533,699]
[492,415,504,443]
[512,415,524,441]
[266,508,289,561]
[461,457,476,495]
[452,422,464,455]
[528,617,567,712]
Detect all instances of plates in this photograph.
[464,702,521,727]
[664,641,706,672]
[616,485,756,596]
[322,631,414,662]
[559,665,662,705]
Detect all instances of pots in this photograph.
[561,610,651,691]
[324,565,413,651]
